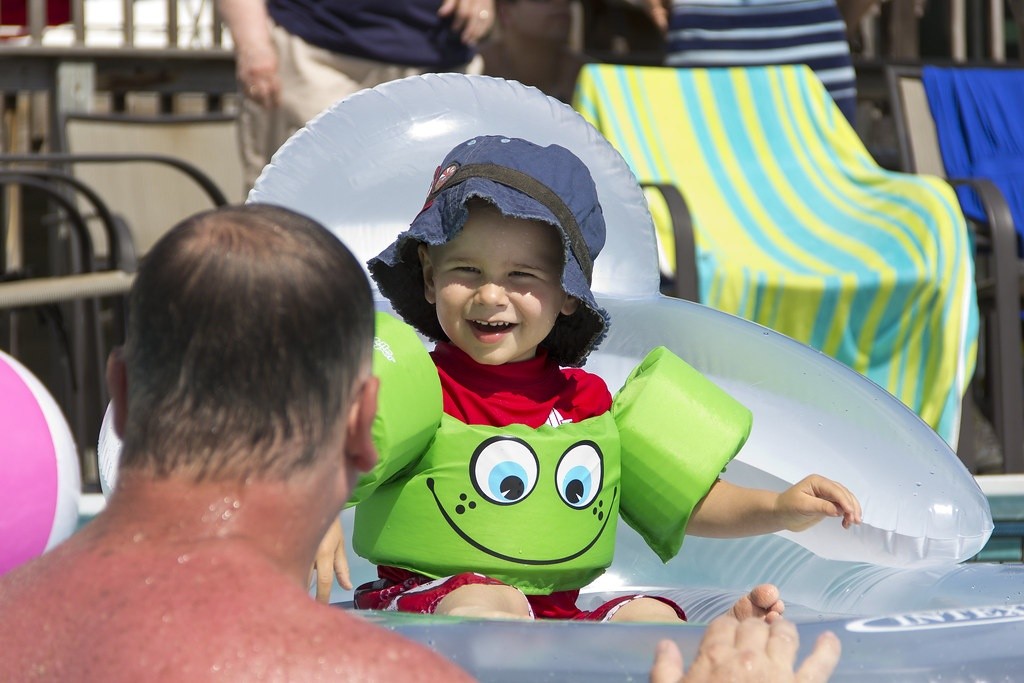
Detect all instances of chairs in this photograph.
[572,59,990,464]
[890,54,1024,475]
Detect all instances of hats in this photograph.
[366,136,612,368]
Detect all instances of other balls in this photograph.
[0,348,83,579]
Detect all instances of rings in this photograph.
[479,10,488,18]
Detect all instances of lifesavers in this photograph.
[335,313,752,595]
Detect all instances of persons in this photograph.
[0,198,847,681]
[211,0,497,217]
[308,133,864,630]
[632,0,859,124]
